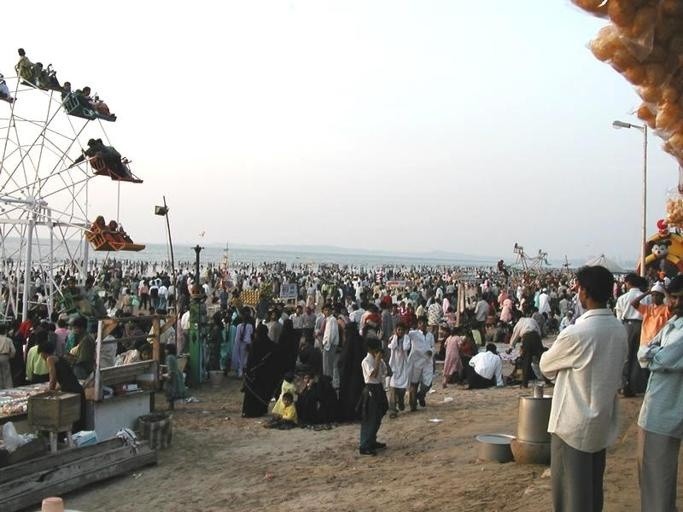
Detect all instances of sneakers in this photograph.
[359,448,374,454]
[371,442,386,448]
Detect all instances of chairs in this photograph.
[159,352,191,384]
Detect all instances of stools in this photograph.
[34,423,75,453]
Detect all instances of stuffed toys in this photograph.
[639,219,683,273]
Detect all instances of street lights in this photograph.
[613,121,648,278]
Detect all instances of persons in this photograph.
[0,244,682,417]
[637,275,682,511]
[0,43,141,251]
[537,265,630,511]
[358,338,392,456]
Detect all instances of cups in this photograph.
[532,383,544,399]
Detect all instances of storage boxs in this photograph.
[63,430,97,449]
[26,390,81,428]
[0,437,46,466]
